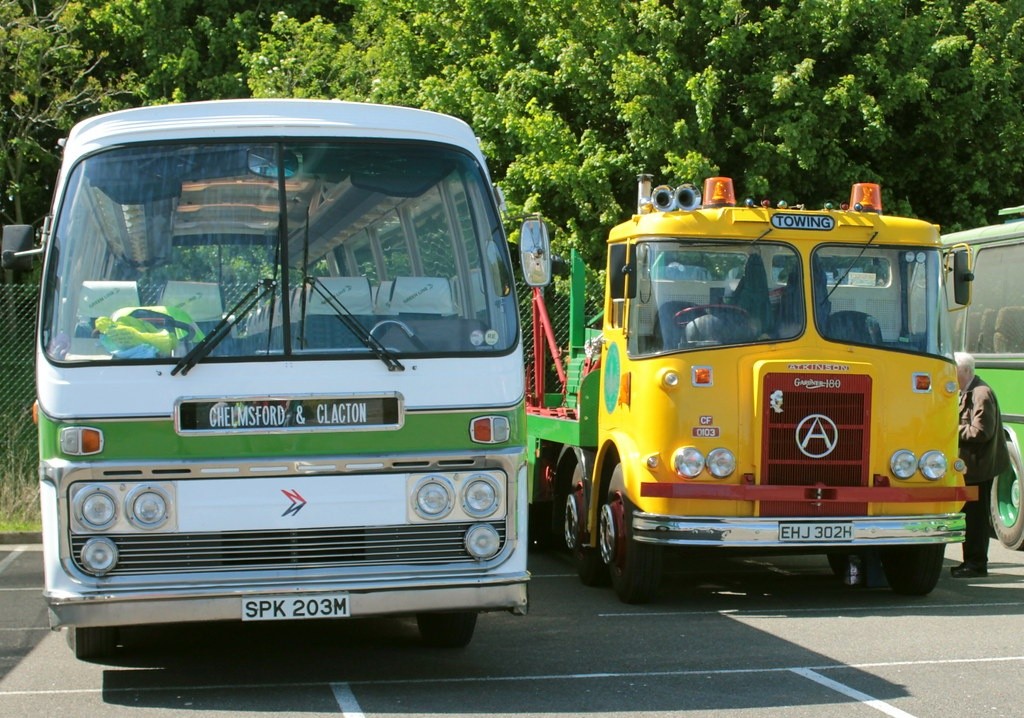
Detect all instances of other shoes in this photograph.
[951,563,988,578]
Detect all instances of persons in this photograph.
[945,352,1013,577]
[682,255,832,343]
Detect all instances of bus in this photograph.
[0,97,553,662]
[910,204,1024,550]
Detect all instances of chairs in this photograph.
[954,306,1024,352]
[655,301,885,349]
[246,273,454,352]
[77,279,235,352]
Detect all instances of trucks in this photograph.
[522,174,977,606]
